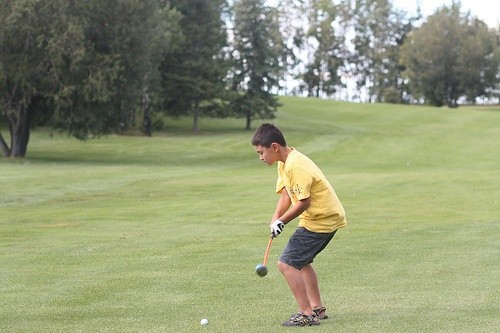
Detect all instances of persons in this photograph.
[252,123,347,325]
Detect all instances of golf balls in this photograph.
[200,318,209,327]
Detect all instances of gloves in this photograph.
[271,219,285,237]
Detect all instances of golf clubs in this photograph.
[255,231,276,277]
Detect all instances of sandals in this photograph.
[282,313,320,326]
[313,307,328,319]
